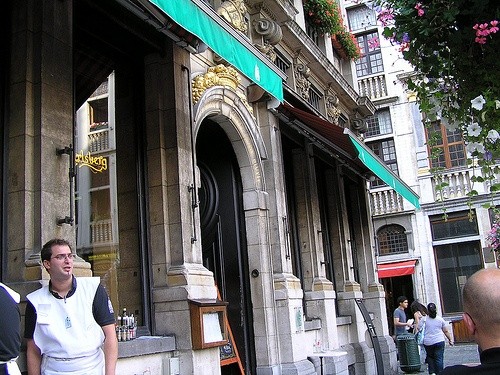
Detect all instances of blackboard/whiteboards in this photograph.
[217,290,240,367]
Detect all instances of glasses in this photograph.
[52,254,78,261]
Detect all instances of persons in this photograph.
[393,296,454,375]
[24,239,120,375]
[0,282,23,375]
[438,267,500,375]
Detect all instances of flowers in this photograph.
[303,0,364,62]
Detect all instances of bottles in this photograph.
[115,308,137,342]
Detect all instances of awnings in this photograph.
[378,259,419,278]
[150,0,289,105]
[281,100,421,208]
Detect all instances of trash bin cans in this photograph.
[396,332,421,374]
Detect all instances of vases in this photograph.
[332,34,347,58]
